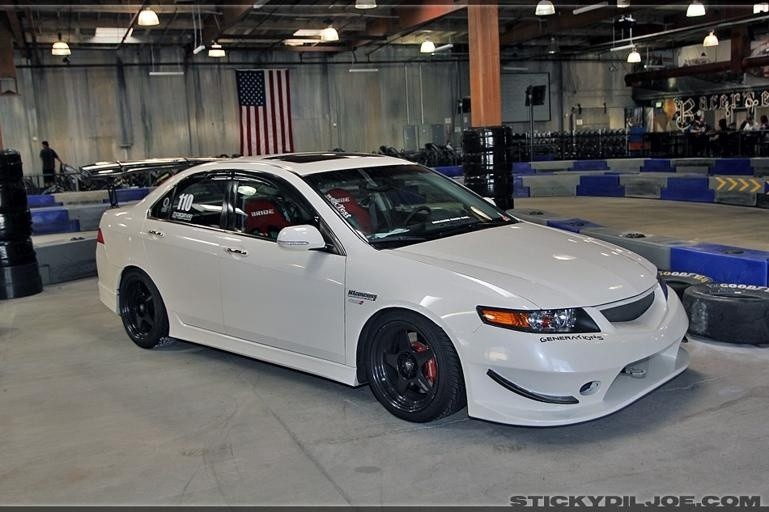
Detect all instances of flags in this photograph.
[236,68,295,156]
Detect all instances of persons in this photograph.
[40,141,64,187]
[719,112,769,130]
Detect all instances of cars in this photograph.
[94,151,691,429]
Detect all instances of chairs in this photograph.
[325,189,371,232]
[241,198,291,238]
